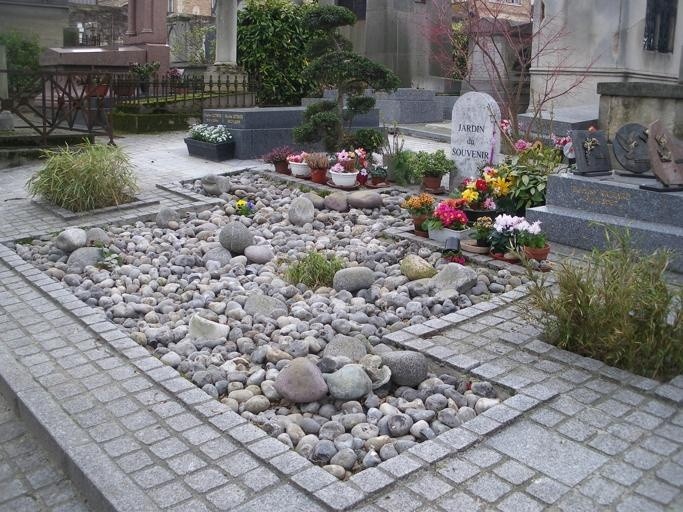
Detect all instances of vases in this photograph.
[327,170,381,189]
[175,87,186,96]
[182,137,234,163]
[490,246,550,271]
[139,82,151,95]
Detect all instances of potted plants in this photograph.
[75,70,109,97]
[409,150,455,189]
[111,70,137,97]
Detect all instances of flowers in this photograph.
[168,66,189,86]
[265,145,387,175]
[132,59,159,81]
[403,164,549,248]
[187,123,232,143]
